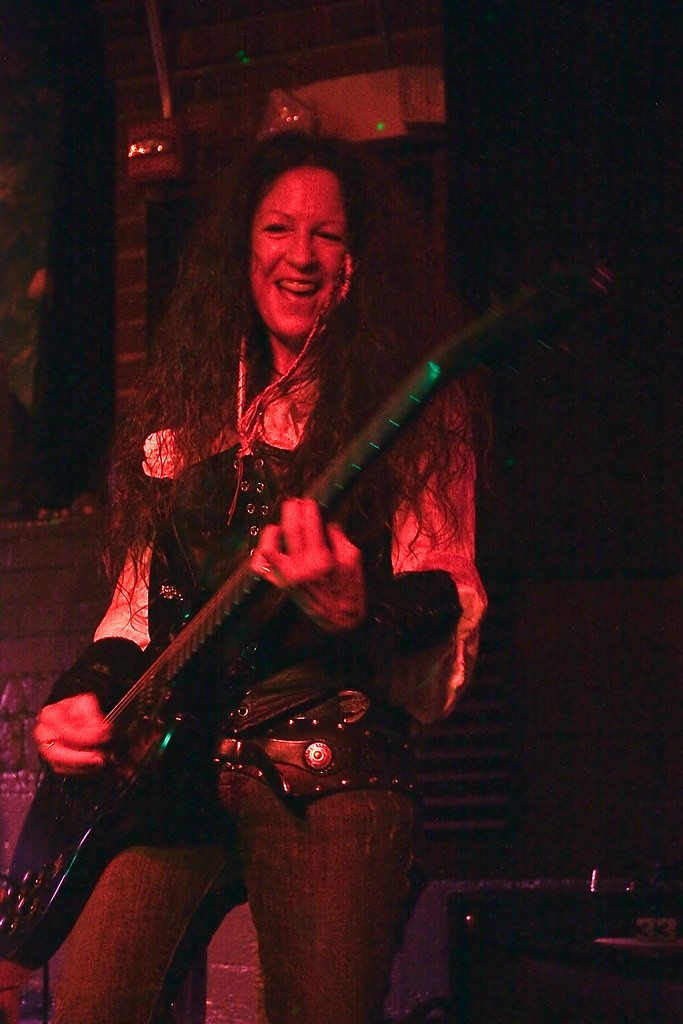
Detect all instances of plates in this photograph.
[595,935,683,966]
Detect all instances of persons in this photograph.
[31,130,484,1022]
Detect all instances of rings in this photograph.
[39,739,61,749]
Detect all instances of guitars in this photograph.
[0,241,633,970]
